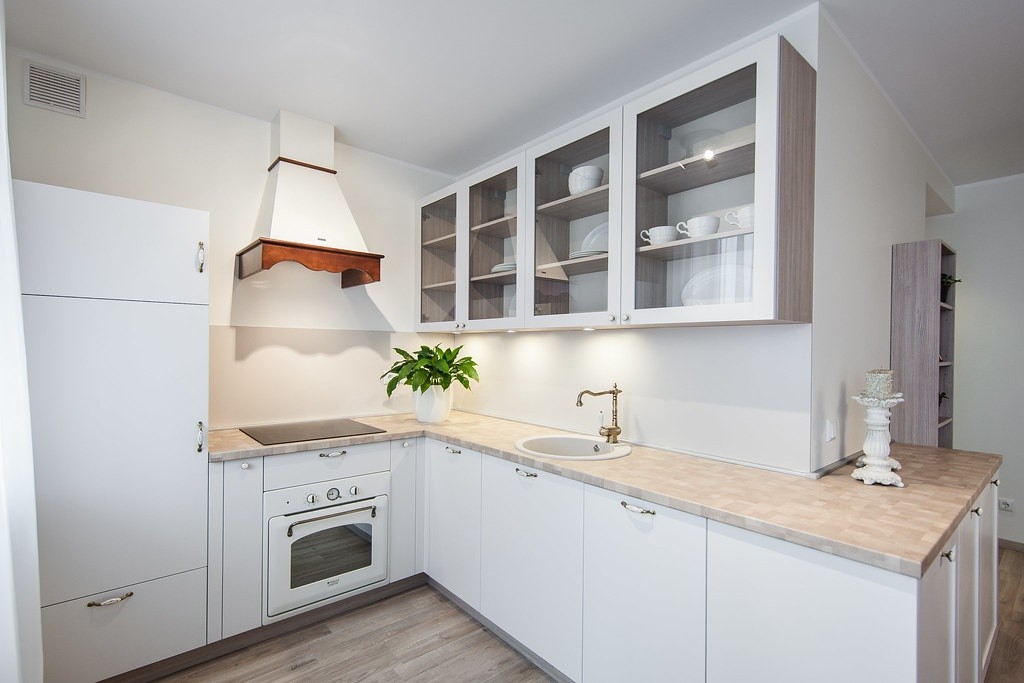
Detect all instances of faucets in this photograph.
[575,382,623,443]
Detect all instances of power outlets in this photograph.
[999,499,1013,512]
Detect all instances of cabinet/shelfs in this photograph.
[8,34,1003,683]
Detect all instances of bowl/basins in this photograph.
[567,165,603,196]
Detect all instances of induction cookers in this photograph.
[238,417,386,447]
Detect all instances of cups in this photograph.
[726,203,754,228]
[639,225,677,245]
[675,215,720,238]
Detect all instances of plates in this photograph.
[509,293,515,317]
[490,262,516,273]
[569,251,608,260]
[680,263,752,306]
[580,222,608,250]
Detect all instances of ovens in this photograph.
[262,470,389,625]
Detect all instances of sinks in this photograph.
[514,434,633,461]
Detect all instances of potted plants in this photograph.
[941,272,961,302]
[380,342,480,424]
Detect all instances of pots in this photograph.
[941,273,962,303]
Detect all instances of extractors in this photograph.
[235,110,385,290]
